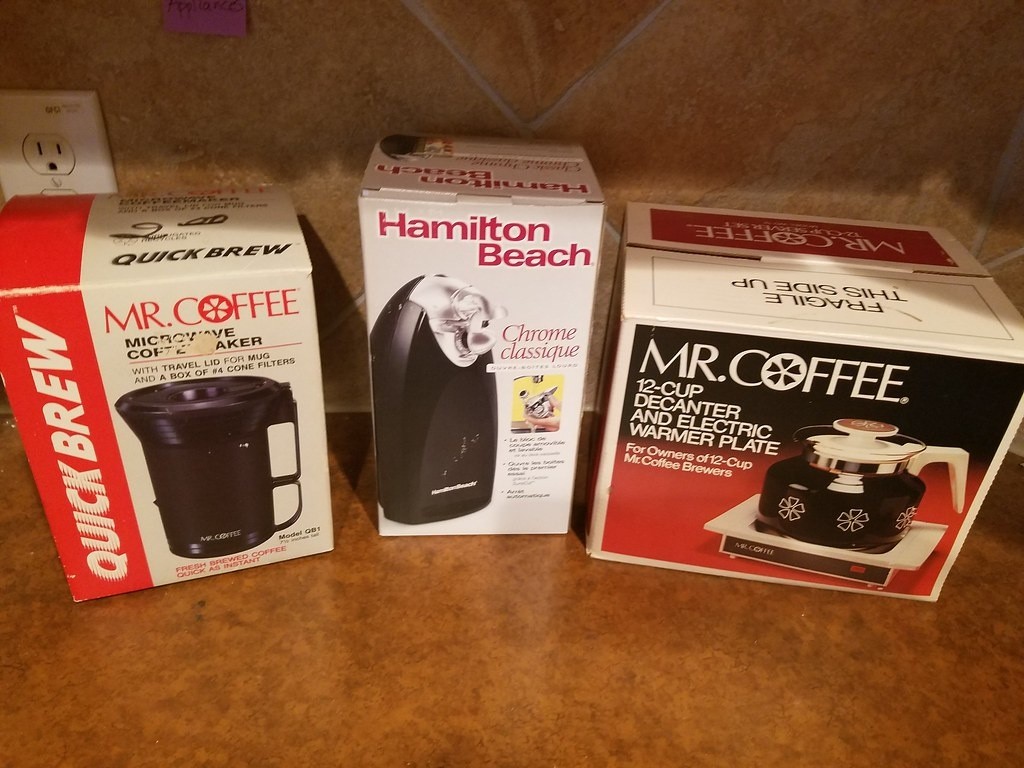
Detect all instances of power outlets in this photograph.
[0,89,118,203]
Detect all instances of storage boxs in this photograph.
[0,185,334,604]
[359,135,607,542]
[586,201,1024,603]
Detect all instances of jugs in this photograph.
[756,418,971,556]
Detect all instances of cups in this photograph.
[116,375,303,559]
